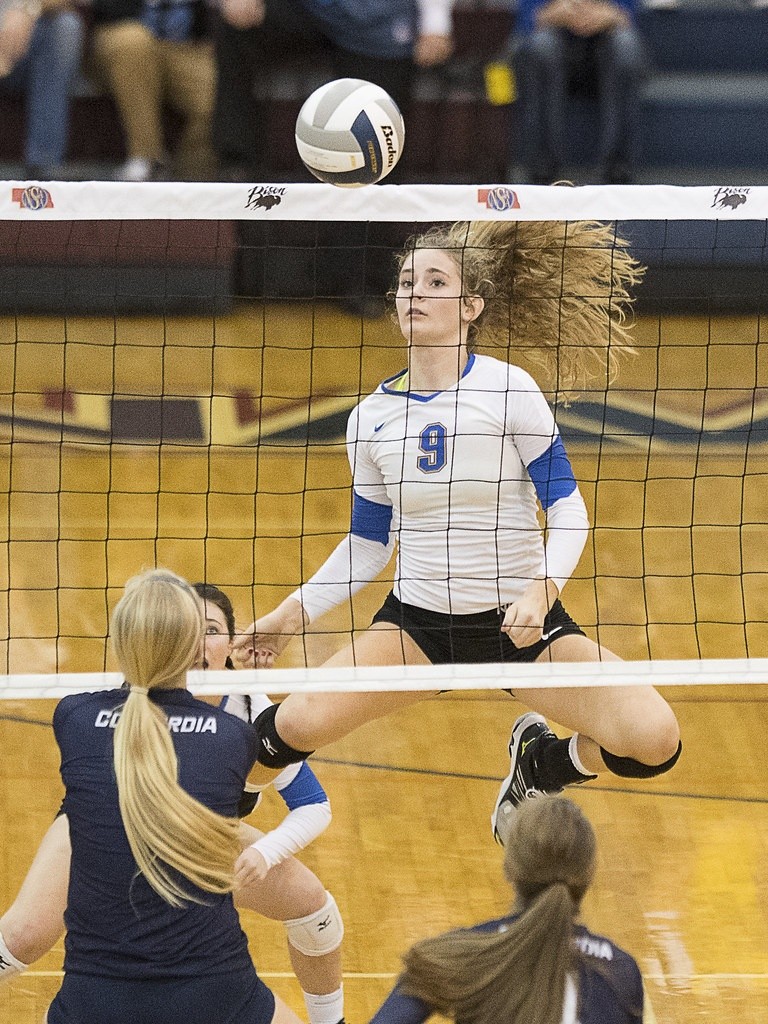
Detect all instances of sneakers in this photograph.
[491,712,560,847]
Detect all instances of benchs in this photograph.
[0,0,768,319]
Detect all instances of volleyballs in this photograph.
[295,78,404,189]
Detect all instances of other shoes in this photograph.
[116,153,167,181]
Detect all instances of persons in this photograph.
[43,568,304,1024]
[0,0,640,181]
[370,795,644,1024]
[0,584,348,1024]
[230,218,683,850]
